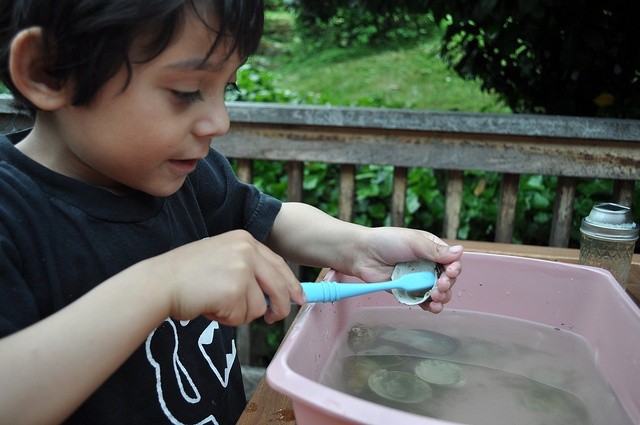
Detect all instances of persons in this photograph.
[2,0,462,425]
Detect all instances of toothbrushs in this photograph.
[254,271,437,303]
